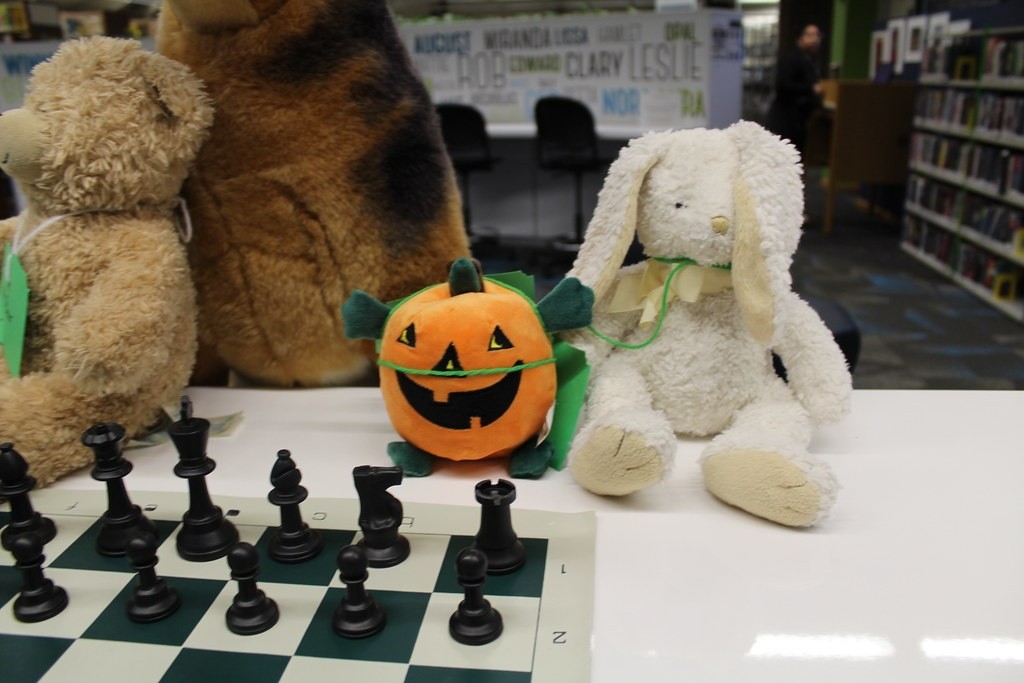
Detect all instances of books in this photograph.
[906,28,1024,301]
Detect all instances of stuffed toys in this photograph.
[0,35,216,491]
[341,258,595,480]
[552,118,853,527]
[156,0,472,389]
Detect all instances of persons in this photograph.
[775,20,827,168]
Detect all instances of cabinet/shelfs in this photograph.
[900,26,1023,323]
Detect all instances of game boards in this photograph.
[0,511,550,683]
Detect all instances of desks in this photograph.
[484,121,664,142]
[0,387,1024,683]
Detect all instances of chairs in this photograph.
[435,101,506,259]
[533,95,619,278]
